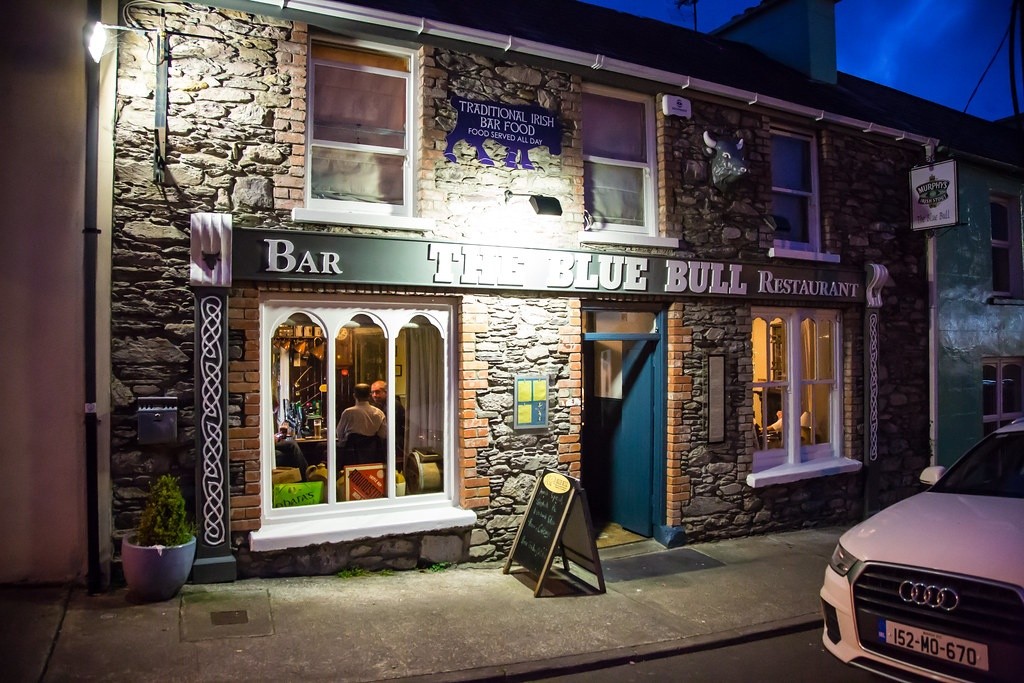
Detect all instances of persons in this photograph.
[273,395,308,471]
[337,383,387,468]
[369,380,406,473]
[759,410,783,449]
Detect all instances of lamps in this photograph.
[504,189,563,217]
[82,19,168,66]
[272,336,326,362]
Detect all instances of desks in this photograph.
[291,437,328,469]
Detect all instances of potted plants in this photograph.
[120,473,201,603]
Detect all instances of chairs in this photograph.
[336,432,385,470]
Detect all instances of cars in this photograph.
[819,419,1024,683]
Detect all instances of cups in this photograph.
[314,419,322,440]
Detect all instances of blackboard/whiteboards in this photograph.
[511,470,577,577]
